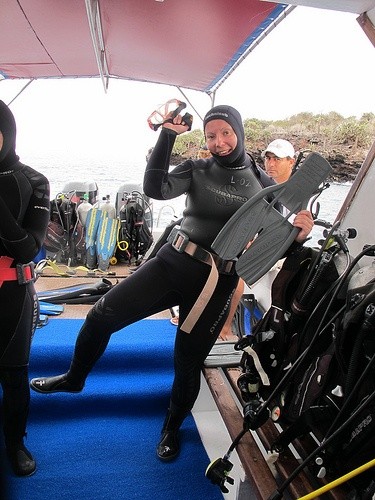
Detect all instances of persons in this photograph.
[31,105,314,461]
[171,138,297,341]
[0,99,50,477]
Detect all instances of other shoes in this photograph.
[157,430,179,460]
[29,373,81,393]
[6,442,37,477]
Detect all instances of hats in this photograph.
[261,140,295,160]
